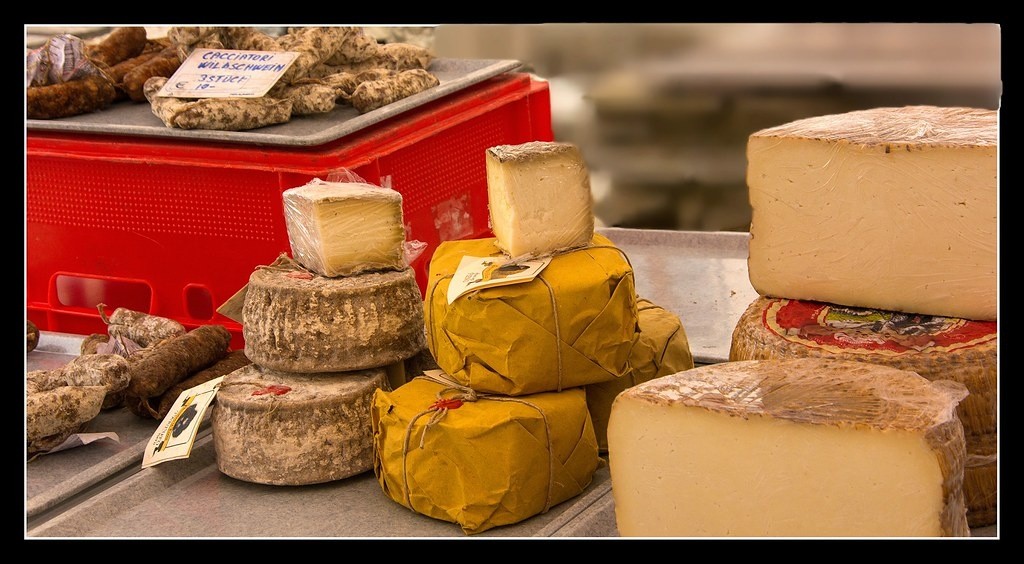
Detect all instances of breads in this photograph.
[207,105,997,537]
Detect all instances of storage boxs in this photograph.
[25,70,555,351]
